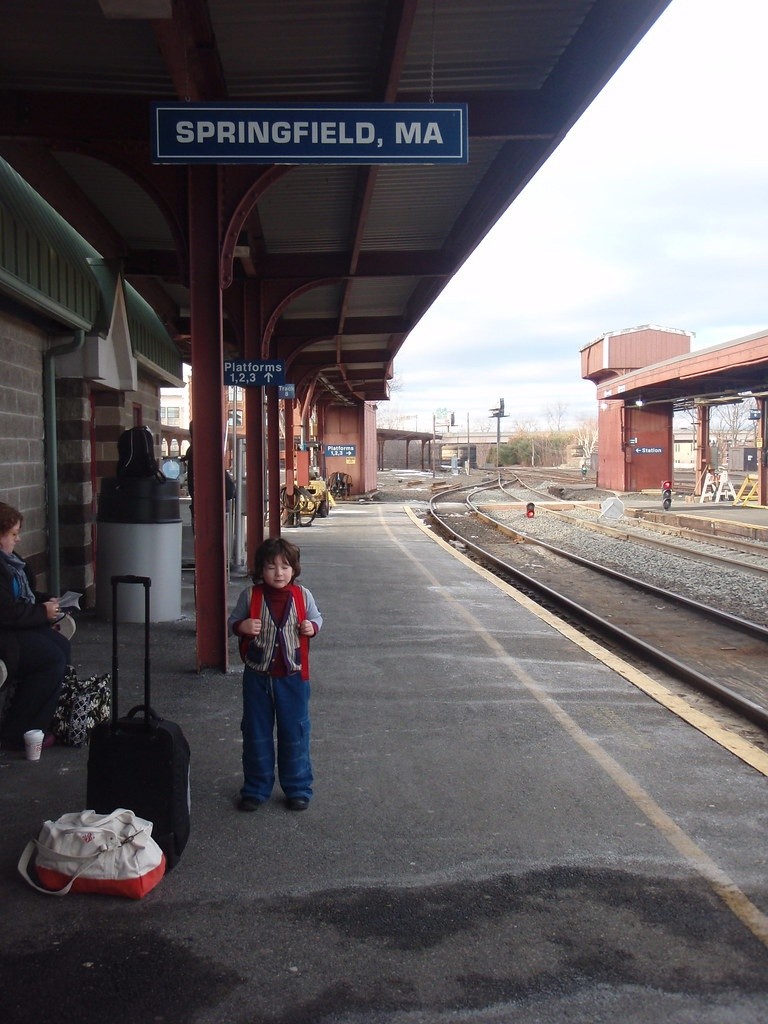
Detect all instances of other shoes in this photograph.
[238,797,260,812]
[287,796,309,810]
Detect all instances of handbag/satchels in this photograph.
[51,666,112,749]
[17,809,167,900]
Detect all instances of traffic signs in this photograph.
[223,358,285,387]
[325,444,357,457]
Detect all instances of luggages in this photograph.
[84,573,191,873]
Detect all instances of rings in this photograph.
[55,608,59,611]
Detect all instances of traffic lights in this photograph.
[526,503,535,518]
[661,480,672,510]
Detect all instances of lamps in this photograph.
[372,405,378,412]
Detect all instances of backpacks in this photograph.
[116,425,167,485]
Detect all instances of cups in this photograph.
[23,729,45,761]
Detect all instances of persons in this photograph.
[227,537,323,813]
[179,420,237,640]
[0,502,70,749]
